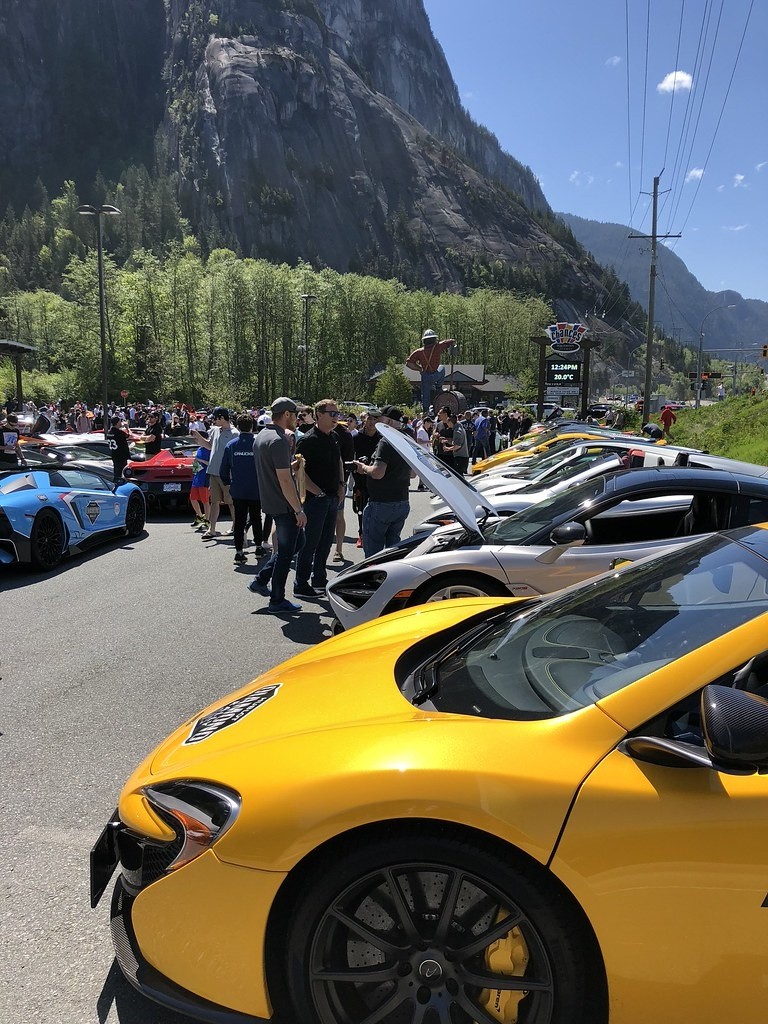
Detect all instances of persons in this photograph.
[0,323,678,566]
[246,400,414,615]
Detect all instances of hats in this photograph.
[207,407,229,419]
[270,397,309,414]
[423,417,435,423]
[368,405,404,423]
[148,411,159,419]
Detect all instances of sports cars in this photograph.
[88,516,767,1024]
[325,380,768,637]
[1,429,220,570]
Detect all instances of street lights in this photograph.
[75,203,123,441]
[298,294,316,406]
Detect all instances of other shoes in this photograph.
[293,582,325,597]
[263,543,270,549]
[234,553,247,564]
[254,546,268,558]
[356,538,362,548]
[333,551,343,562]
[311,577,329,589]
[243,531,249,547]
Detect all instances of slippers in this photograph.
[219,529,234,536]
[202,532,217,539]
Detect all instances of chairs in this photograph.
[671,491,718,537]
[731,649,768,700]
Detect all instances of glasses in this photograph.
[149,415,155,418]
[323,410,341,418]
[7,421,17,427]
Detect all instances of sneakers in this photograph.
[195,520,210,533]
[267,598,302,613]
[191,514,207,527]
[247,580,271,596]
